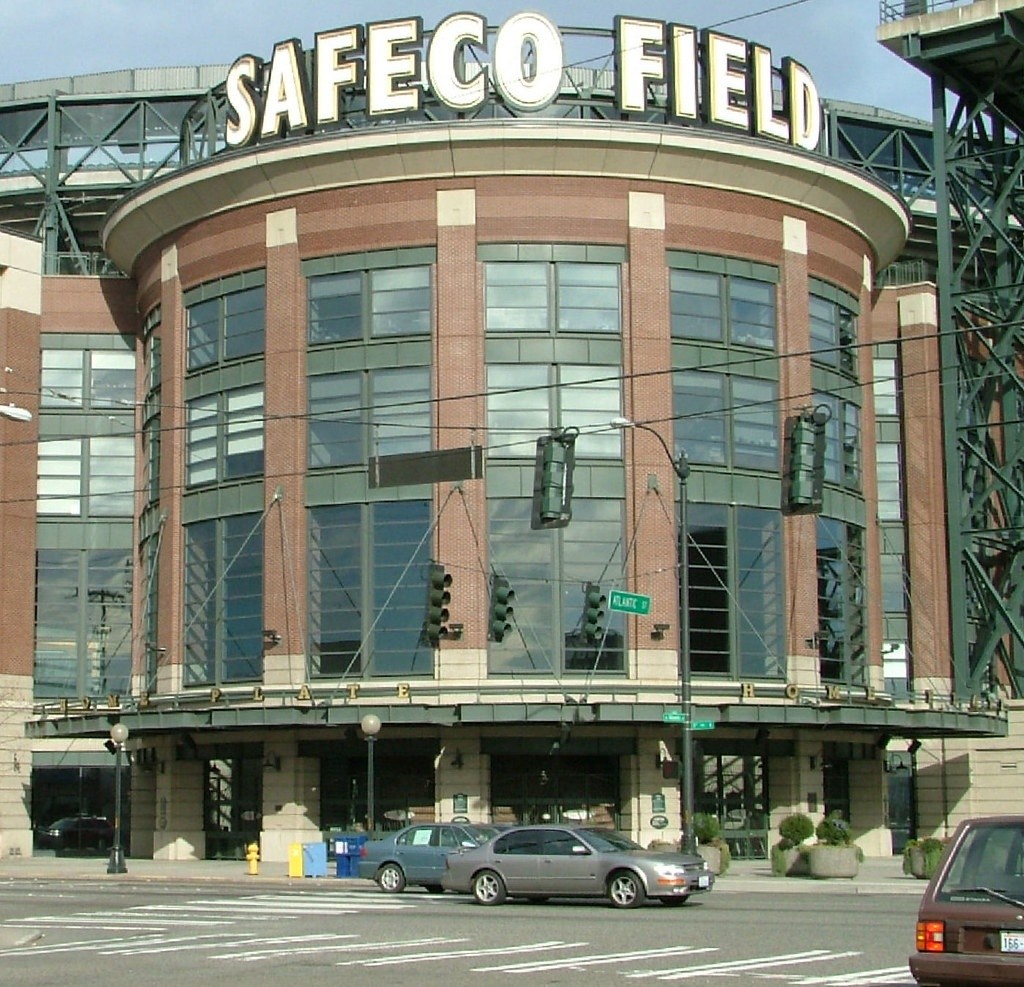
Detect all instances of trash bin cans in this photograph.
[287,842,327,878]
[334,833,368,878]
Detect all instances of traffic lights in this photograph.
[579,581,607,643]
[485,576,515,643]
[421,564,454,646]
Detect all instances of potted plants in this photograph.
[693,812,731,875]
[807,813,864,879]
[647,840,681,854]
[770,813,816,877]
[903,837,944,879]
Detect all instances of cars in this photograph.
[908,815,1024,987]
[357,823,499,896]
[462,823,517,842]
[39,818,115,851]
[441,825,715,909]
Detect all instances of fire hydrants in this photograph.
[245,845,260,875]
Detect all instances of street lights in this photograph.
[361,714,382,841]
[609,418,705,862]
[107,723,129,874]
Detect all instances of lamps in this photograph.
[261,630,281,646]
[807,631,832,645]
[451,748,464,769]
[260,750,281,773]
[908,740,922,754]
[181,734,197,752]
[754,724,771,746]
[875,733,892,750]
[652,623,670,640]
[883,755,908,774]
[655,749,667,768]
[209,764,220,773]
[344,728,358,741]
[104,741,118,755]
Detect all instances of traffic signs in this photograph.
[607,589,651,617]
